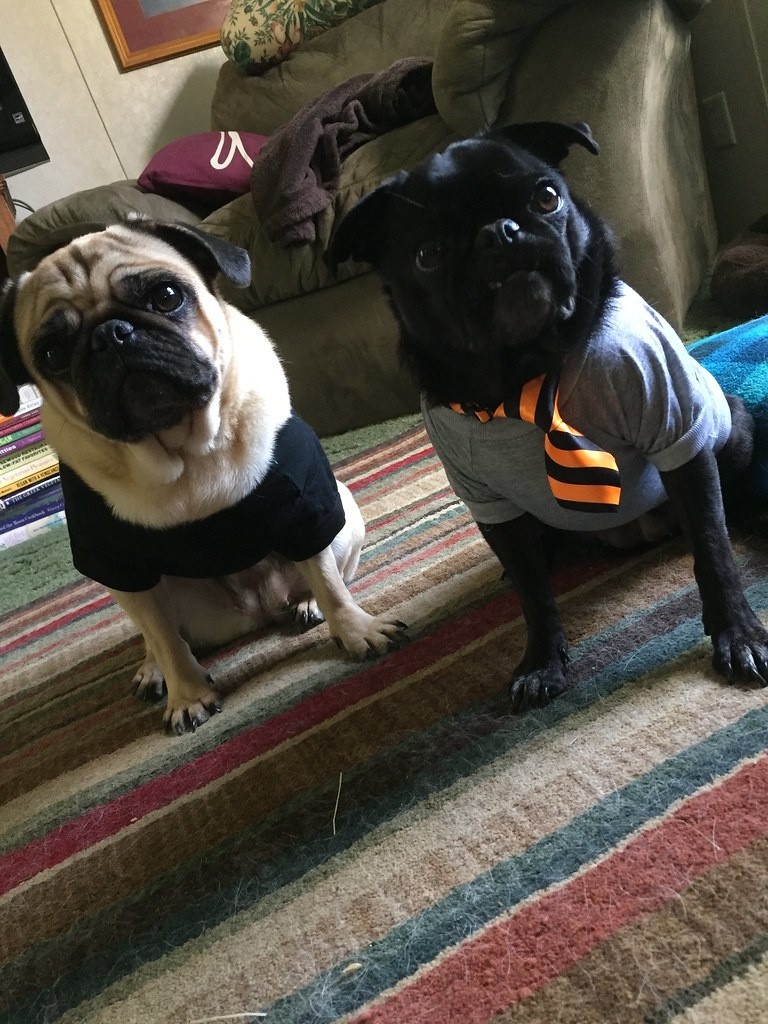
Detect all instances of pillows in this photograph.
[431,0,571,144]
[134,128,277,207]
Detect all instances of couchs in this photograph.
[6,0,723,447]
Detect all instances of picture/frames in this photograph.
[89,0,236,76]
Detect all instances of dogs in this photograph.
[0,121,768,734]
